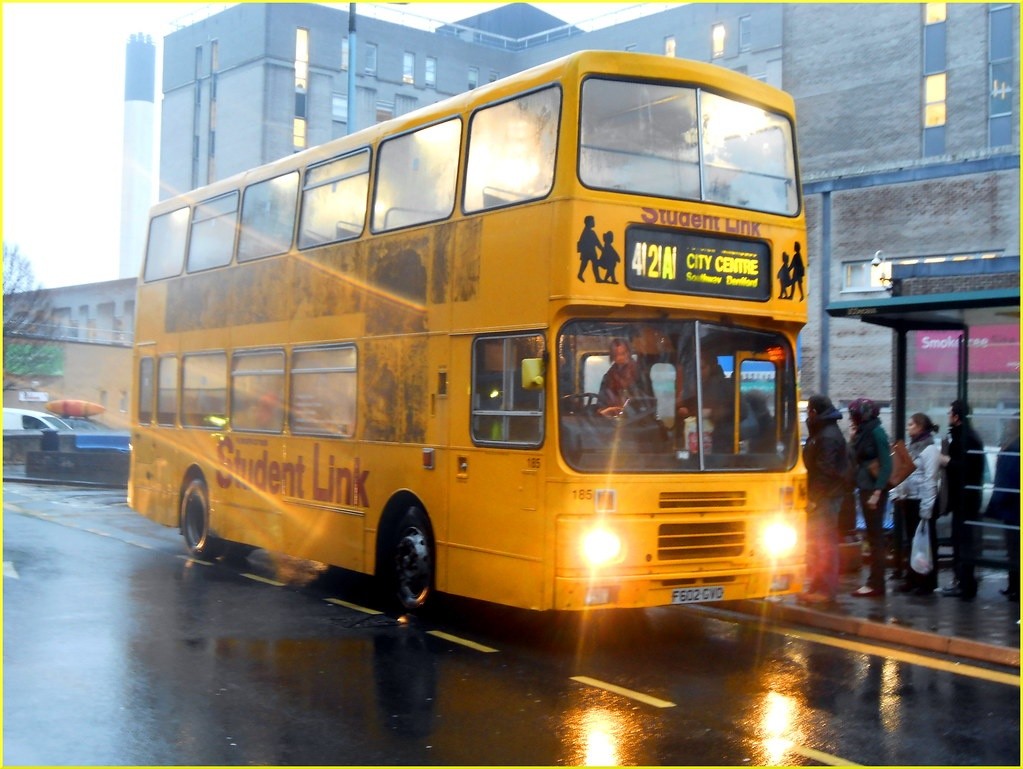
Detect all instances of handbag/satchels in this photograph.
[683,416,714,455]
[867,439,917,491]
[910,519,934,575]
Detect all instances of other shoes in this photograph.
[1008,592,1019,600]
[852,583,885,597]
[795,591,833,603]
[897,582,917,592]
[913,585,934,595]
[944,587,962,596]
[999,586,1015,595]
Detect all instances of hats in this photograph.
[849,397,879,430]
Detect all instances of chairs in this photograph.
[298,188,533,247]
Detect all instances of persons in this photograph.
[800,393,1020,597]
[590,338,779,455]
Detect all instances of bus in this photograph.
[123,49,814,629]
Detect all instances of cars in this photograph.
[1,407,131,452]
